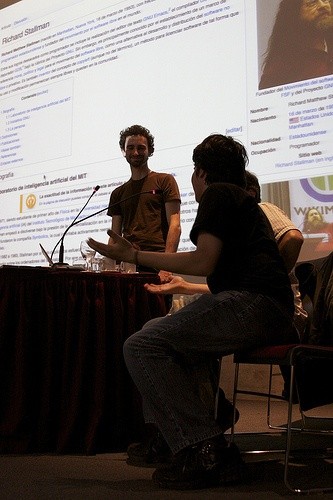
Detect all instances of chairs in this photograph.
[230,250,333,496]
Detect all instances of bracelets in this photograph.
[133,249,141,266]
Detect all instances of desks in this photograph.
[0,265,173,456]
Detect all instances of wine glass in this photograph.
[80,240,96,272]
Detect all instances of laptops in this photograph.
[39,243,53,264]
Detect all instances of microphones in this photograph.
[49,185,161,267]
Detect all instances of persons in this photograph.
[257,0,333,90]
[85,124,304,487]
[302,208,333,251]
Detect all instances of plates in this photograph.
[121,272,139,274]
[100,270,117,272]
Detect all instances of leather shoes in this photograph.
[152,433,244,491]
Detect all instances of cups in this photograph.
[100,258,115,269]
[73,257,86,269]
[92,257,99,272]
[120,261,137,272]
